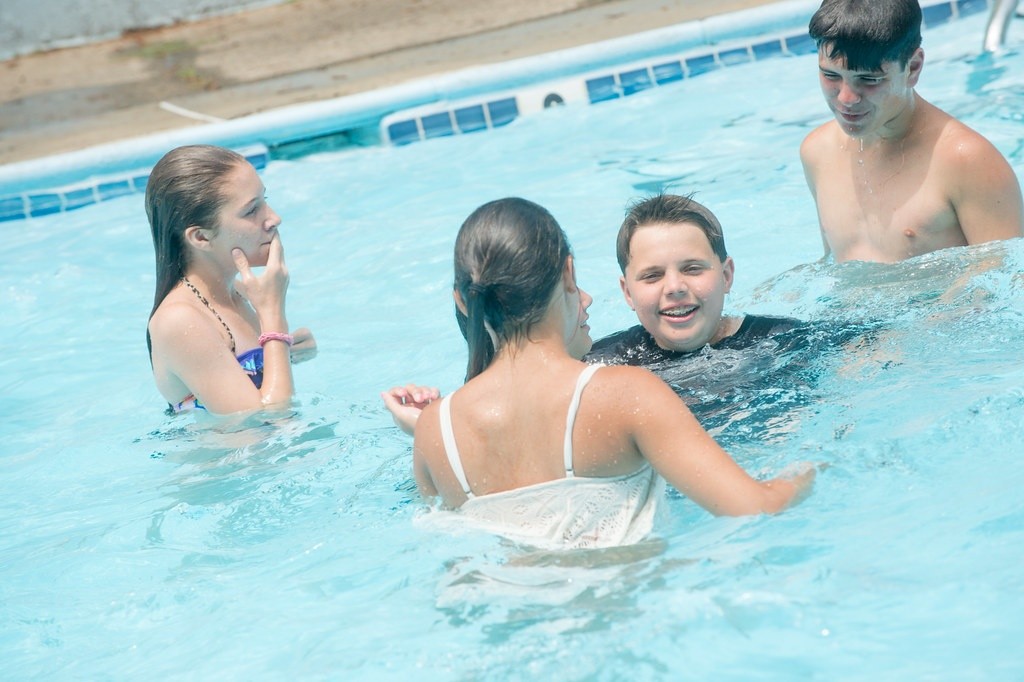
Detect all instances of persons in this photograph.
[381,195,818,551]
[800,0,1024,266]
[146,146,318,416]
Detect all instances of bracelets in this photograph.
[258,332,294,347]
